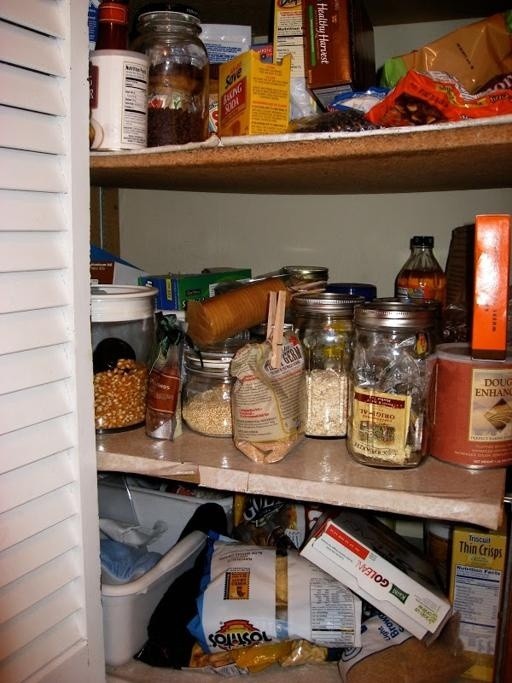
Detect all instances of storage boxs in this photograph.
[99,484,210,666]
[98,471,234,535]
[448,525,508,683]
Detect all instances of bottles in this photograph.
[395,236,449,302]
[429,341,512,469]
[91,281,159,435]
[292,291,366,440]
[137,4,209,146]
[84,2,149,156]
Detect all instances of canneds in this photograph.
[281,265,512,471]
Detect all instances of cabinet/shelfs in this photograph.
[89,119,512,683]
